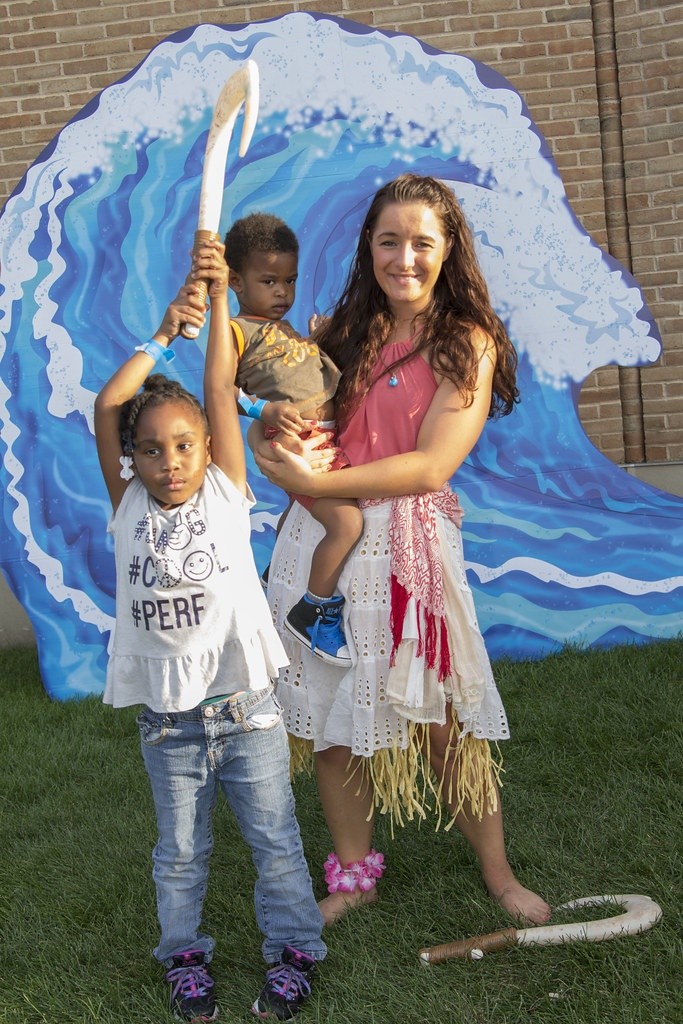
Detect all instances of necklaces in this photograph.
[377,329,425,387]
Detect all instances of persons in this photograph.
[245,169,550,930]
[222,210,361,669]
[95,235,327,1023]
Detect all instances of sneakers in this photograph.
[164,950,218,1023]
[283,592,353,668]
[252,945,317,1023]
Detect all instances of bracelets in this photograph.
[133,338,177,366]
[235,386,269,421]
